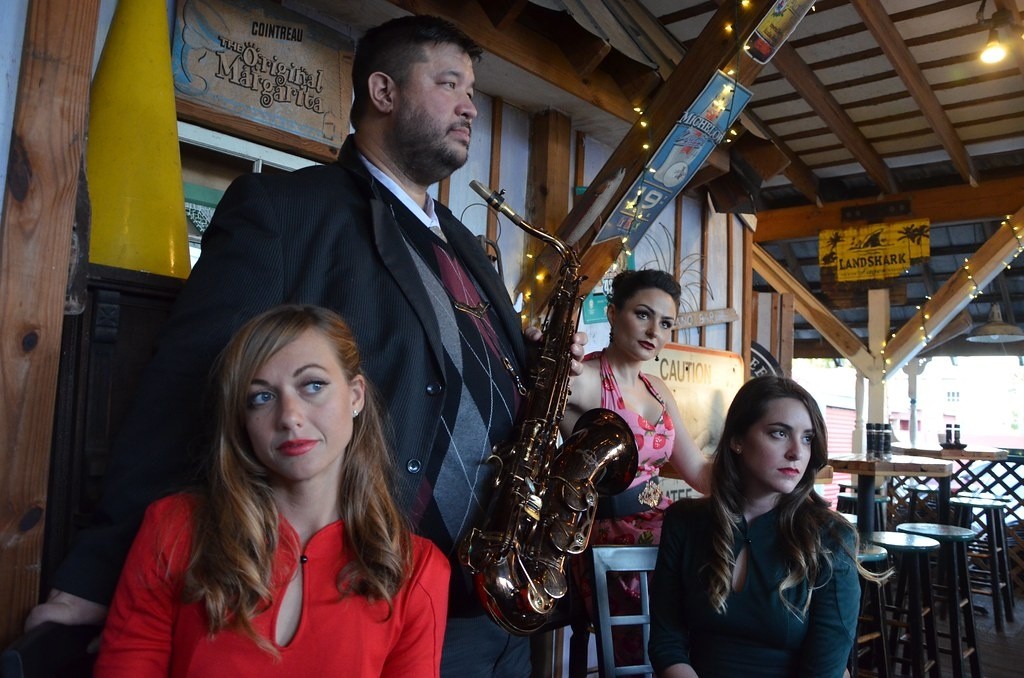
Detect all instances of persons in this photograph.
[24,16,588,677]
[94,304,451,678]
[558,269,715,604]
[647,375,863,678]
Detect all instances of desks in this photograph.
[890,441,1008,616]
[658,454,834,484]
[827,452,953,672]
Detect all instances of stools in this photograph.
[836,493,891,532]
[896,522,984,678]
[836,482,883,495]
[955,490,1015,607]
[902,484,939,566]
[939,497,1015,637]
[846,544,892,678]
[869,530,941,678]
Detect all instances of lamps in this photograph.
[965,299,1024,344]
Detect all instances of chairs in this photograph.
[589,543,658,678]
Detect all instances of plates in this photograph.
[940,443,967,449]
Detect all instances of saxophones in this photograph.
[458,181,641,635]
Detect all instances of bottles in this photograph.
[884,424,892,460]
[866,423,874,461]
[874,423,884,461]
[946,424,952,443]
[954,424,960,443]
